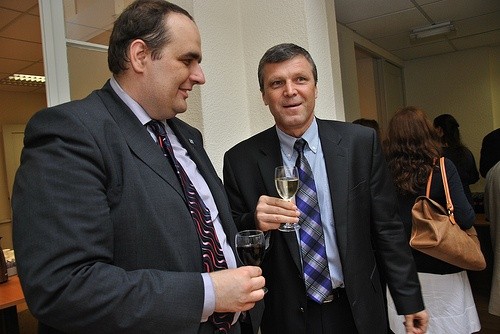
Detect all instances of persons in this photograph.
[433,114,480,209]
[480,128,500,178]
[485,160,500,316]
[222,44,428,334]
[386,106,482,334]
[11,0,265,334]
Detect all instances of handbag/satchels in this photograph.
[409,156,487,271]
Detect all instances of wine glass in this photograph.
[275,166,301,232]
[235,230,268,294]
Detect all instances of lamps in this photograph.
[409,21,457,40]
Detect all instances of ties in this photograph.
[291,138,333,304]
[147,120,236,334]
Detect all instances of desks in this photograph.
[0,274,38,334]
